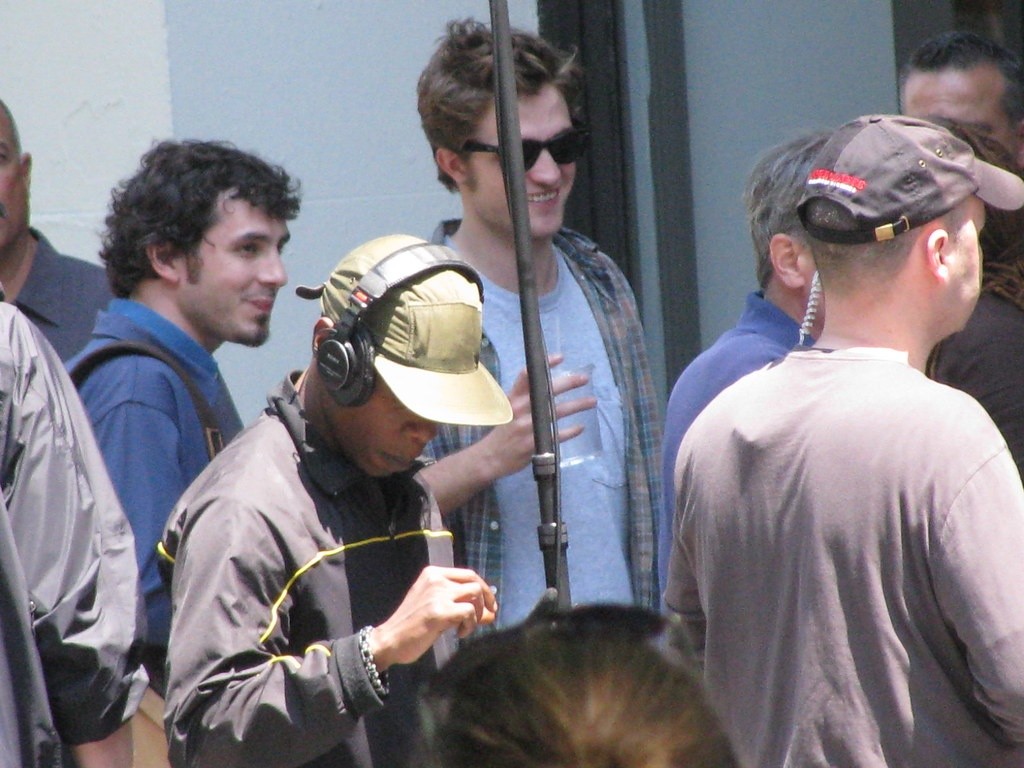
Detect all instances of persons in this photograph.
[157,234,498,768]
[420,604,742,768]
[658,30,1024,768]
[62,138,304,700]
[415,16,663,643]
[0,99,150,768]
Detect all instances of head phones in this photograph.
[315,241,484,409]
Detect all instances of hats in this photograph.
[795,115,1024,244]
[319,235,513,426]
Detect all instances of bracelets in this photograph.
[356,625,390,699]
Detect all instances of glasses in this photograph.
[425,604,704,767]
[456,116,590,173]
